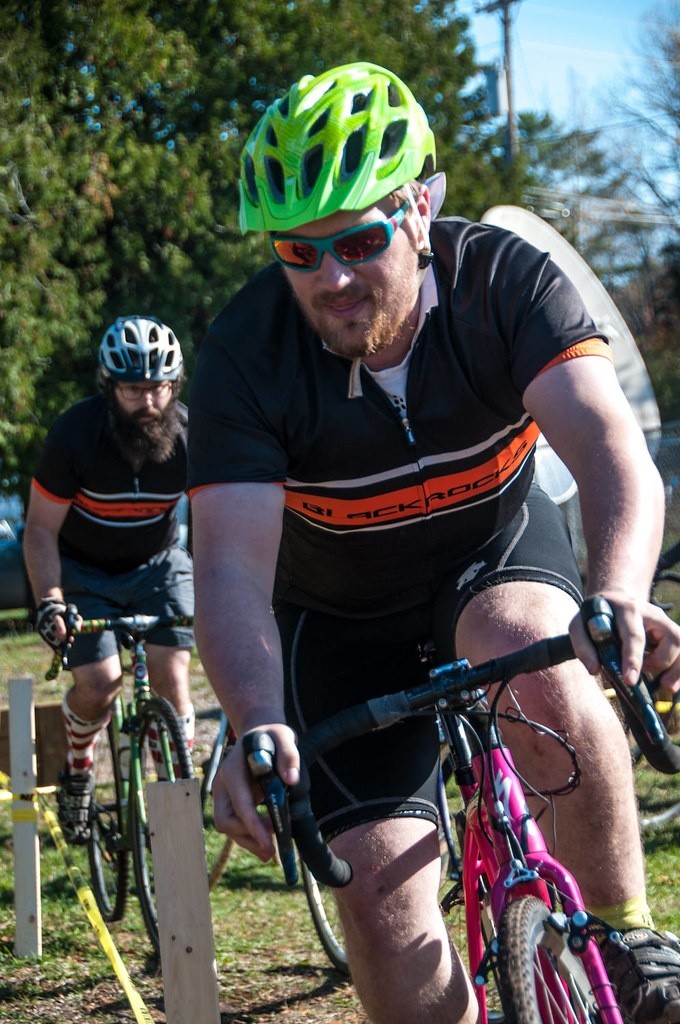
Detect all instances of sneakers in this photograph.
[601,927,680,1024]
[58,763,94,845]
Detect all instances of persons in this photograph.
[21,312,199,847]
[186,62,680,1024]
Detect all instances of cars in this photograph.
[0,479,192,625]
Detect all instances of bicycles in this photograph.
[197,531,680,891]
[243,594,680,1024]
[41,600,199,955]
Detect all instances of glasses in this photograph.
[267,201,410,271]
[115,381,173,400]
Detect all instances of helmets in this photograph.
[238,60,436,237]
[98,315,184,381]
